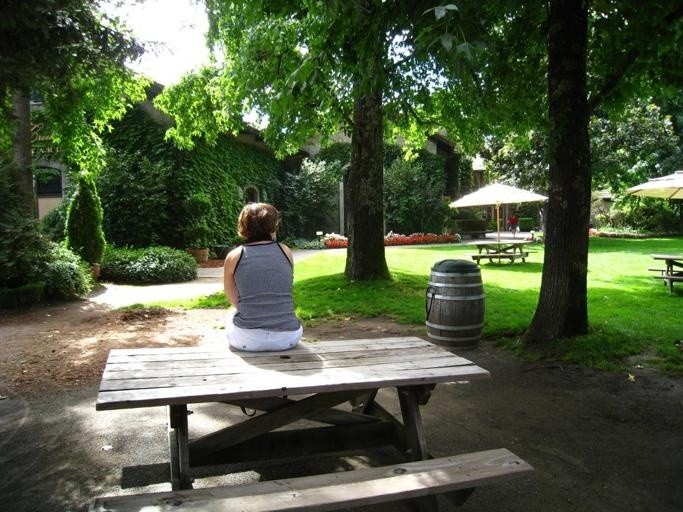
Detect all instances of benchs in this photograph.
[472,250,538,265]
[93,446,534,512]
[647,267,683,294]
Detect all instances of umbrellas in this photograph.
[627,170,683,200]
[447,182,548,263]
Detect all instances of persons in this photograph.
[223,200,304,353]
[508,213,519,239]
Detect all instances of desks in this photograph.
[467,240,533,265]
[94,335,492,490]
[649,254,683,294]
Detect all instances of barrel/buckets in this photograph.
[423,265,485,347]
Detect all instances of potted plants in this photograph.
[61,168,106,282]
[181,194,236,263]
